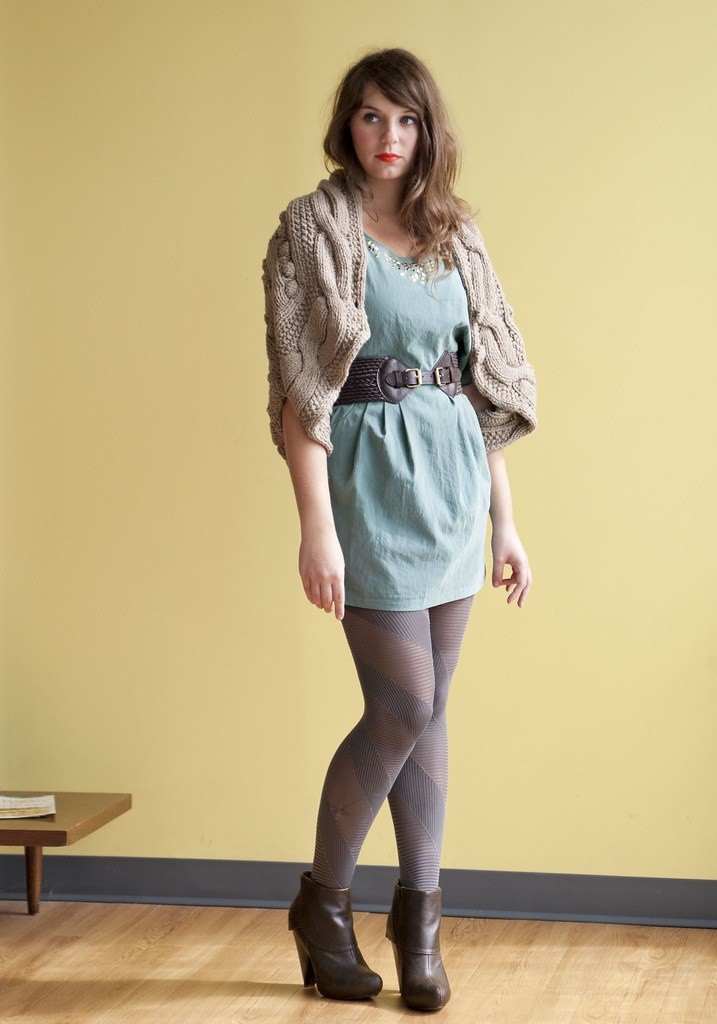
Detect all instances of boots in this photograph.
[385,879,451,1010]
[288,871,383,1000]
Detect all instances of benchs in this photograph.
[0,791,133,916]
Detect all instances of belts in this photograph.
[332,349,463,405]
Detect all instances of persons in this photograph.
[261,47,538,1013]
